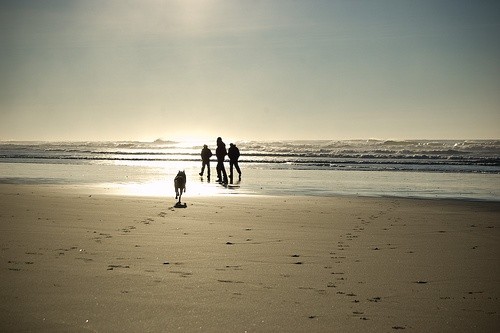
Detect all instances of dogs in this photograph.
[174,170,186,203]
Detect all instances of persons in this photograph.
[215,136,228,184]
[198,144,212,178]
[227,142,242,178]
